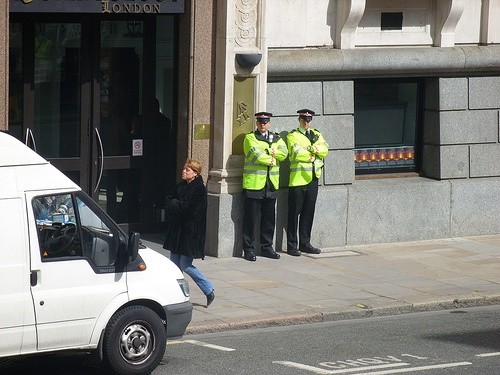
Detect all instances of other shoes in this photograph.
[207,288,215,306]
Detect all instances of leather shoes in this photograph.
[287,250,301,255]
[261,249,280,259]
[299,244,321,254]
[244,251,256,261]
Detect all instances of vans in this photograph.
[1,131,197,375]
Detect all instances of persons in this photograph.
[287,109,328,256]
[242,112,289,261]
[162,160,214,307]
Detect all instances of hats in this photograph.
[254,112,273,123]
[297,109,315,120]
